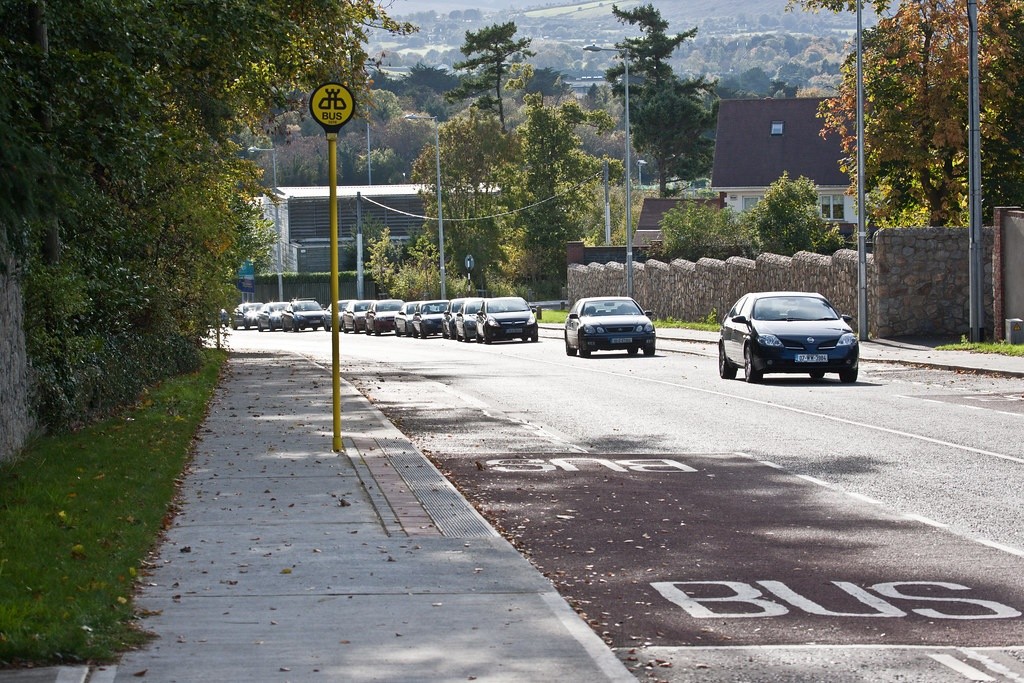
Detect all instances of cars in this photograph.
[341,300,374,334]
[231,302,255,330]
[282,298,326,332]
[364,299,405,336]
[717,291,860,383]
[455,299,484,343]
[394,300,424,337]
[256,301,292,333]
[412,300,449,339]
[476,296,539,343]
[321,300,351,331]
[442,298,465,339]
[238,302,265,330]
[208,309,228,328]
[564,296,656,356]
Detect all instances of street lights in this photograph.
[583,43,636,299]
[404,114,446,302]
[248,144,284,301]
